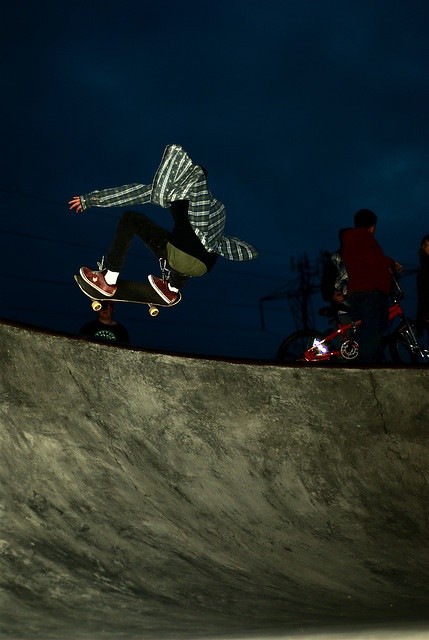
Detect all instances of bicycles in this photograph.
[276,261,429,365]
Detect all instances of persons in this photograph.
[341,211,399,328]
[66,143,259,304]
[320,229,403,306]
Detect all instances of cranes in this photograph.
[258,252,418,331]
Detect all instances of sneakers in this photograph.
[79,255,117,297]
[148,274,178,305]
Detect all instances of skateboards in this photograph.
[74,273,182,317]
